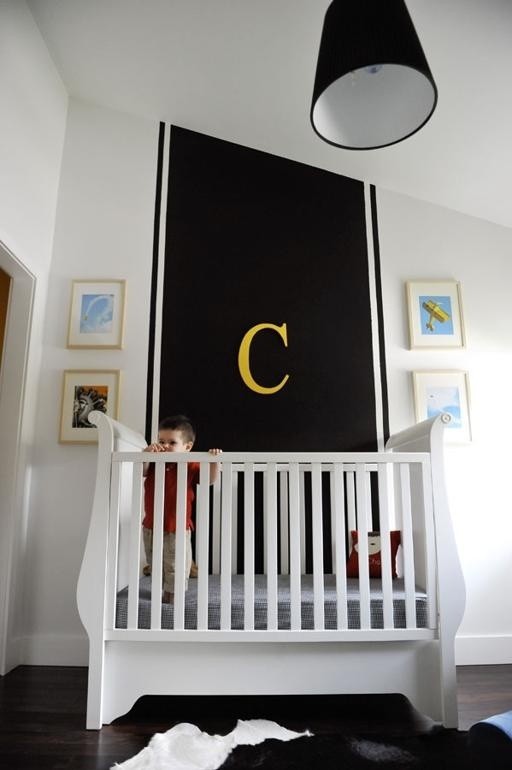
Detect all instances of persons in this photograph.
[140,414,223,604]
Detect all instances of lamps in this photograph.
[311,0,437,149]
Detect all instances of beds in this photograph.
[75,410,467,730]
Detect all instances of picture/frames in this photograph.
[405,281,467,350]
[57,369,120,445]
[66,279,127,348]
[413,370,475,446]
